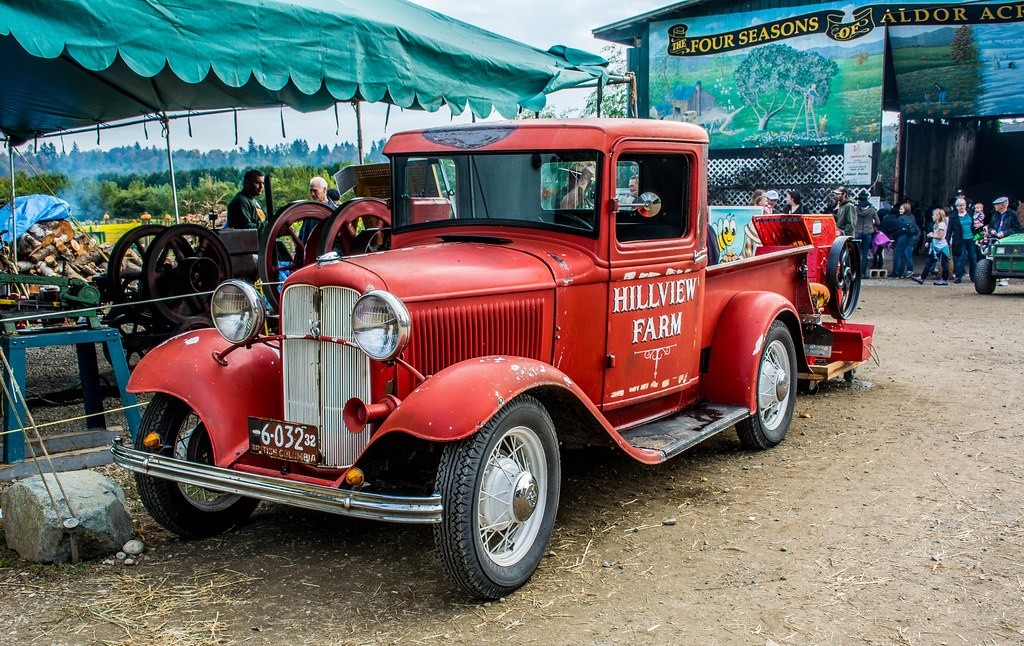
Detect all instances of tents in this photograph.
[0,0,638,266]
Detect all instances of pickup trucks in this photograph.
[109,118,822,600]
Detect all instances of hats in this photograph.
[956,189,964,195]
[993,197,1008,203]
[833,186,848,193]
[559,163,595,181]
[857,194,868,201]
[766,190,778,200]
[327,189,339,200]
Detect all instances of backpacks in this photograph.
[890,215,919,235]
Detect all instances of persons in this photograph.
[551,162,595,209]
[750,190,810,214]
[227,169,293,262]
[628,175,639,210]
[824,185,1021,285]
[327,189,342,204]
[295,177,337,253]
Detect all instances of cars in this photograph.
[974,232,1024,294]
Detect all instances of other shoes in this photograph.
[900,275,904,279]
[999,281,1008,286]
[954,278,961,283]
[887,275,899,280]
[933,278,948,285]
[906,271,914,277]
[911,275,924,284]
